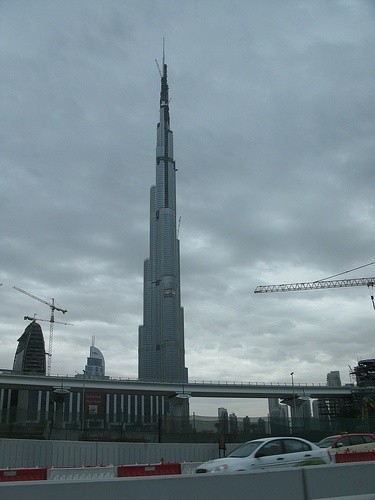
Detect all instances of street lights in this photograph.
[290,371,299,436]
[81,369,87,437]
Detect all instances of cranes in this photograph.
[255,261,375,312]
[10,285,74,378]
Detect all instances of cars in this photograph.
[195,437,329,473]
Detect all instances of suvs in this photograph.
[316,432,375,464]
